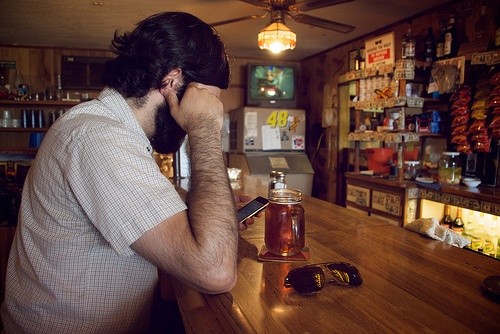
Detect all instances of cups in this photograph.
[265,187,305,257]
[0,108,64,128]
[226,166,242,205]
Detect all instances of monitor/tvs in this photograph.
[246,63,297,109]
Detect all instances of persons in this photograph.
[0,11,262,334]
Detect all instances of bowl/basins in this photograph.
[462,178,481,188]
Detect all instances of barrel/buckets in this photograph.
[365,147,393,175]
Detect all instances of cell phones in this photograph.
[236,196,269,223]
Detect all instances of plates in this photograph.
[416,177,435,184]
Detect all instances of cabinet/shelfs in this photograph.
[0,98,80,133]
[342,59,439,229]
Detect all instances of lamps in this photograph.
[258,10,297,54]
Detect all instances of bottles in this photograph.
[274,171,287,189]
[462,208,500,258]
[14,69,23,88]
[451,207,464,235]
[403,160,420,180]
[438,151,461,186]
[439,204,454,229]
[354,47,365,70]
[400,13,457,60]
[267,170,278,190]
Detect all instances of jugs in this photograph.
[30,132,44,147]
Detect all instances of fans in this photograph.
[206,0,356,34]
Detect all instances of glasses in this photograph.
[283,261,364,294]
